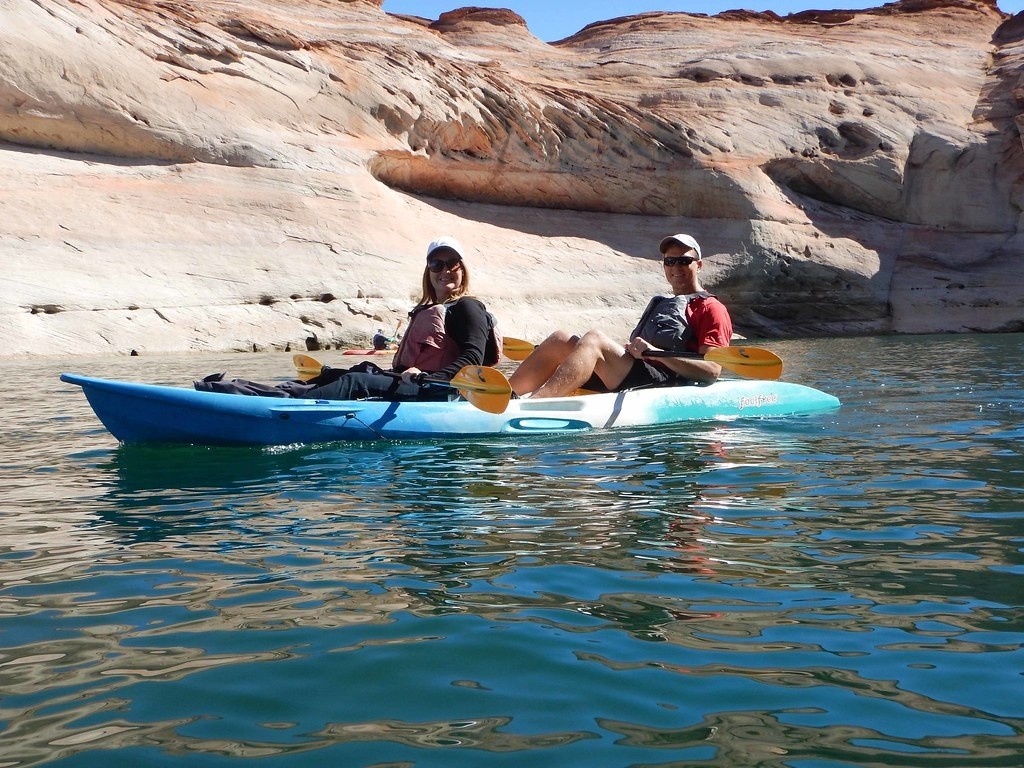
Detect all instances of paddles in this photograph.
[501,334,784,383]
[292,353,512,414]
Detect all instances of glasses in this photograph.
[664,256,698,266]
[428,258,461,272]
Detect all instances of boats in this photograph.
[58,371,843,447]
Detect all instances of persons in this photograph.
[372,329,404,352]
[299,237,500,402]
[503,234,733,398]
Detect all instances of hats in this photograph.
[660,234,701,260]
[426,237,463,259]
